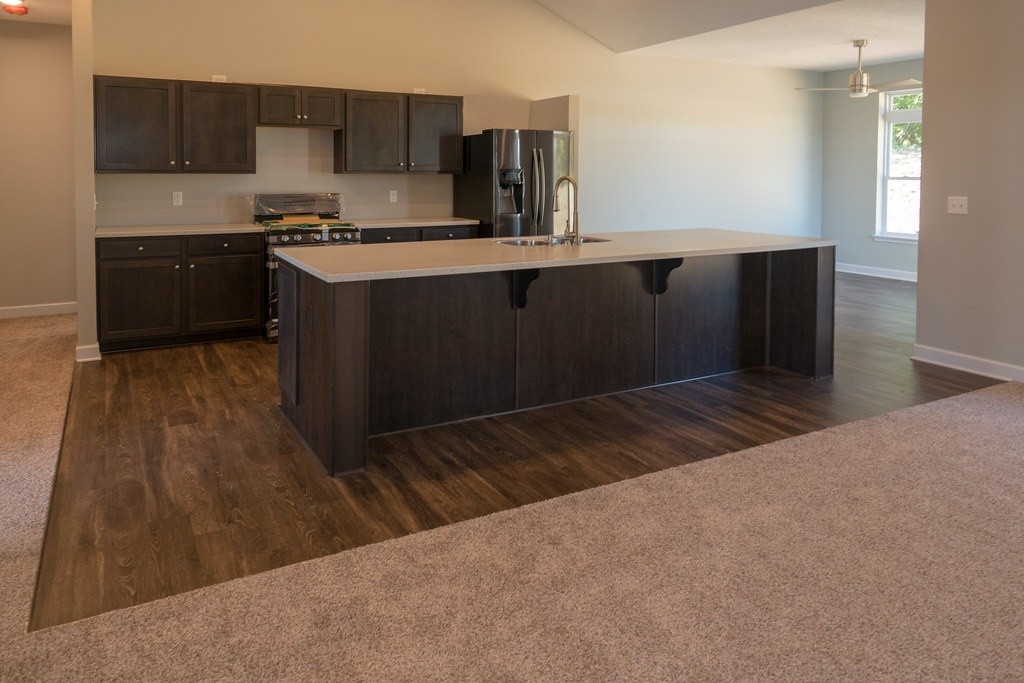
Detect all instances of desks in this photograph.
[275,228,838,477]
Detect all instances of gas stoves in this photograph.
[252,193,361,245]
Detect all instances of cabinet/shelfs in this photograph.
[361,225,478,244]
[95,232,265,353]
[93,75,463,174]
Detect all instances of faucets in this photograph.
[551,176,579,245]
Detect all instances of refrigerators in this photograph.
[453,129,577,238]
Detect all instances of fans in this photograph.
[794,40,923,97]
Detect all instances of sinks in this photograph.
[497,236,612,247]
